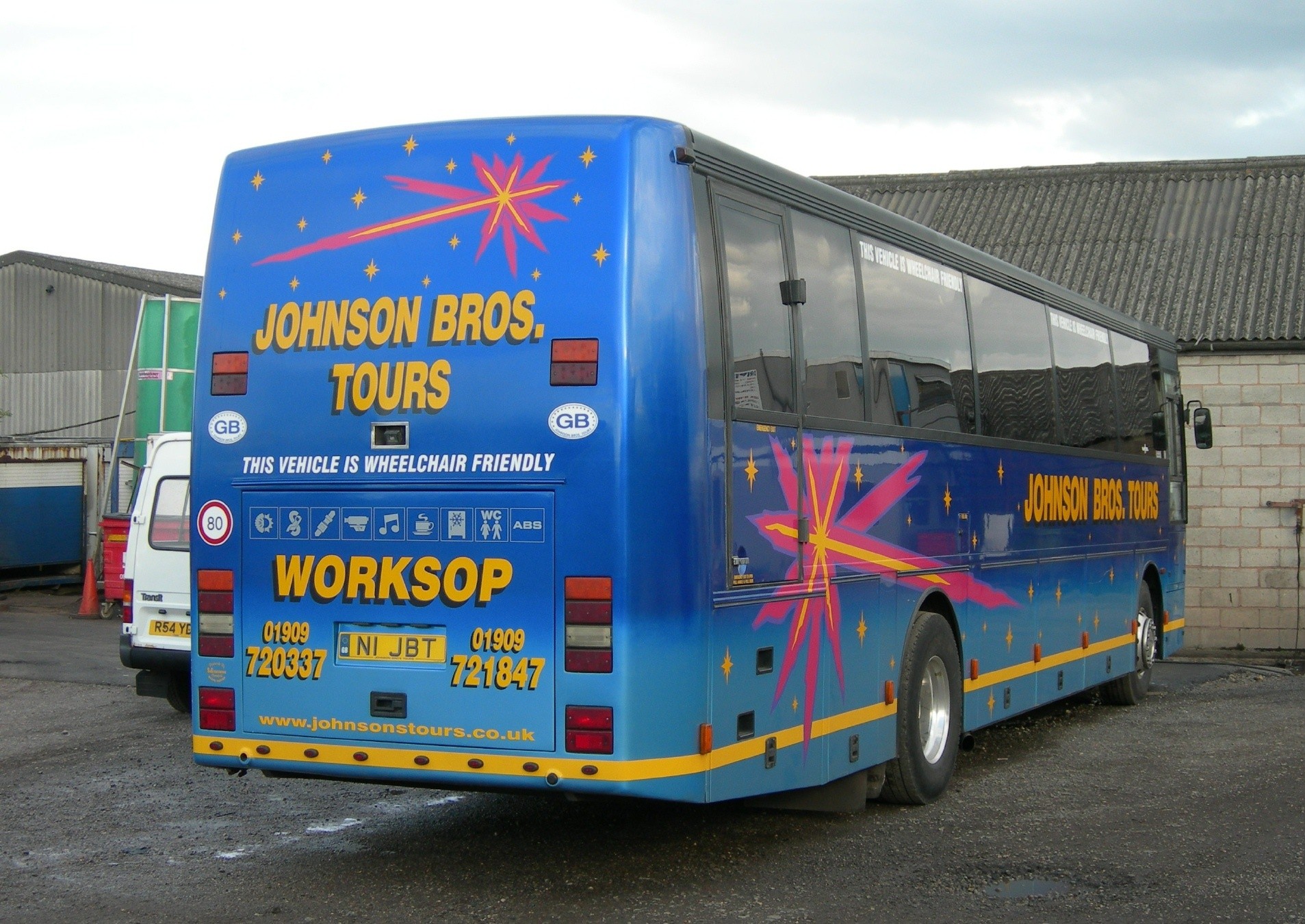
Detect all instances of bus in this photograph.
[189,113,1213,817]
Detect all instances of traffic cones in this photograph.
[71,558,102,620]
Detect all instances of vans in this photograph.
[119,432,191,713]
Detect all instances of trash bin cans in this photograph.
[97,513,131,600]
